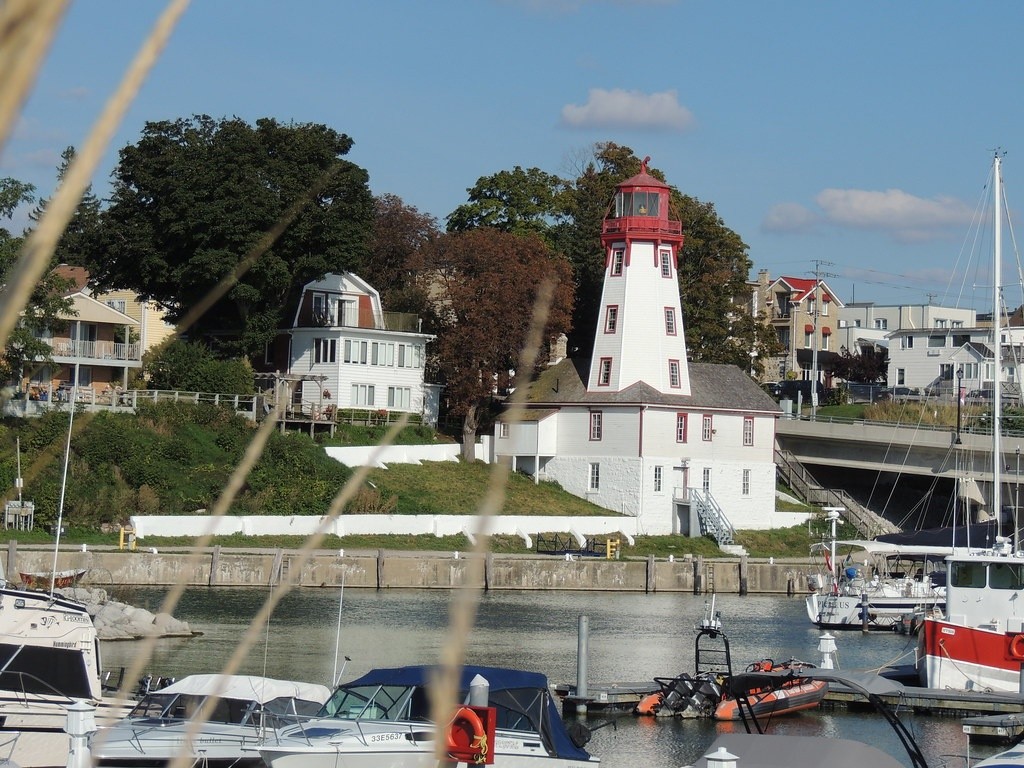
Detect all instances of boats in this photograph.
[1,584,167,768]
[240,661,619,768]
[804,151,1024,634]
[633,588,830,723]
[89,673,340,767]
[909,286,1024,699]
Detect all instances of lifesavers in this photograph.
[447,707,485,759]
[1010,634,1024,658]
